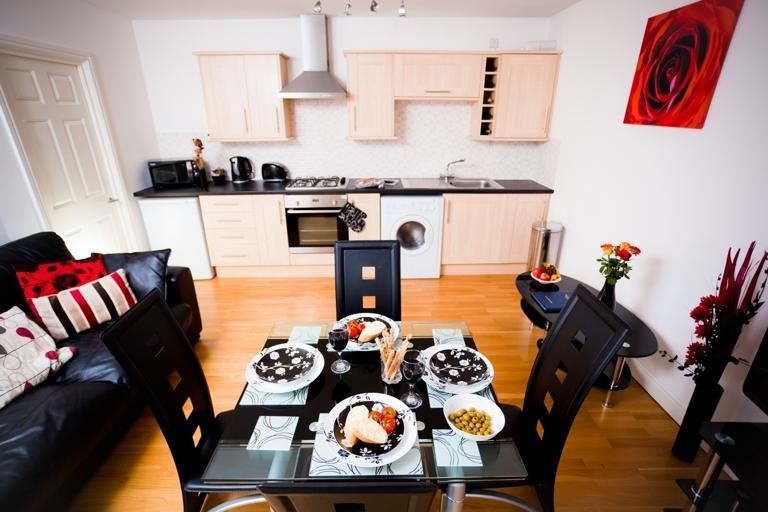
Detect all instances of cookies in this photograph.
[373,326,406,381]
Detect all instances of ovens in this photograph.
[285,208,348,254]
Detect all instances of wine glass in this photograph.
[399,350,425,409]
[327,323,351,374]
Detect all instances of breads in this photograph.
[344,404,369,447]
[353,416,388,444]
[358,320,387,342]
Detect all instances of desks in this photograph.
[688,422,768,512]
[515,268,659,408]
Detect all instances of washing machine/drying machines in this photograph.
[380,177,442,279]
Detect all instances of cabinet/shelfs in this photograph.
[194,51,294,144]
[344,50,562,141]
[199,194,381,278]
[440,193,551,278]
[381,194,444,280]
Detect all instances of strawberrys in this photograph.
[345,319,365,338]
[368,406,396,432]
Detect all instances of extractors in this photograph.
[279,13,347,100]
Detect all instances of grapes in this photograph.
[546,264,557,276]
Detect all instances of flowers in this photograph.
[659,239,768,385]
[599,242,641,282]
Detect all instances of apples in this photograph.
[532,264,550,281]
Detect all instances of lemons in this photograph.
[551,273,560,281]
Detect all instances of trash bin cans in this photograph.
[526,220,564,272]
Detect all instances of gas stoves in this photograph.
[283,176,349,208]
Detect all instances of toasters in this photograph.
[261,163,288,182]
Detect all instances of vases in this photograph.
[672,384,724,463]
[595,279,614,312]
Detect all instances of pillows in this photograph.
[16,253,108,323]
[32,268,138,342]
[102,248,171,302]
[0,304,77,410]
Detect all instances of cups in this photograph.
[211,172,227,187]
[381,346,402,384]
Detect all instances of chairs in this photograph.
[439,285,628,512]
[98,287,276,512]
[334,241,401,323]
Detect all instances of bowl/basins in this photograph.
[442,394,505,442]
[530,269,562,284]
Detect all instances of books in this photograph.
[530,291,573,313]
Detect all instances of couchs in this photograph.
[0,231,201,511]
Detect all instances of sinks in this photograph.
[444,176,505,191]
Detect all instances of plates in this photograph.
[417,344,495,394]
[245,341,326,394]
[324,392,417,467]
[332,312,401,351]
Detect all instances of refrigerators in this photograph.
[137,195,214,282]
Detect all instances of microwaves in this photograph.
[148,159,207,191]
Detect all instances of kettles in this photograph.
[229,157,252,183]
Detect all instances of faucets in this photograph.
[444,156,466,173]
